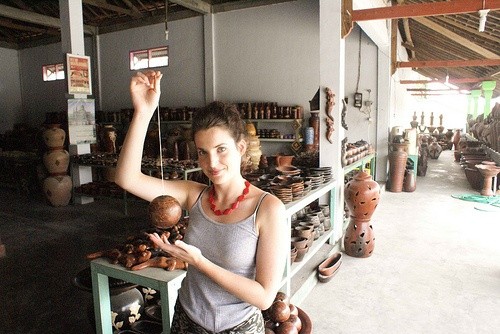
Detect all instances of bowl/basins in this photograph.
[291,222,315,266]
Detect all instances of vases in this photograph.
[429,143,442,159]
[43,123,65,146]
[388,143,416,193]
[304,110,319,150]
[289,204,330,264]
[344,216,375,257]
[475,164,500,196]
[100,125,117,152]
[344,175,380,219]
[272,155,295,166]
[43,173,72,207]
[42,148,70,175]
[71,267,144,334]
[167,124,197,159]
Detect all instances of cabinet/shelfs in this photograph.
[70,163,212,216]
[97,118,305,142]
[283,179,335,299]
[0,156,42,193]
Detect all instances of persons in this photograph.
[114,69,290,334]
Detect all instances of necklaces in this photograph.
[208,180,250,215]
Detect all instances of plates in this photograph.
[256,165,332,203]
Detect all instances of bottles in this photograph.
[305,201,331,239]
